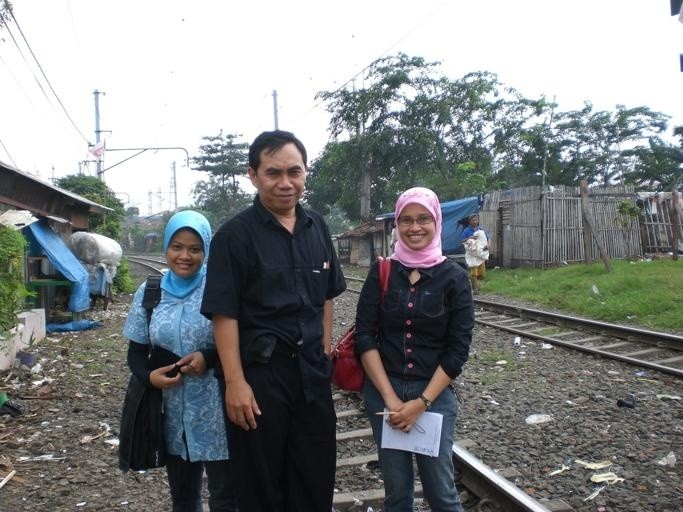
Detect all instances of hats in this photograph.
[468,214,478,221]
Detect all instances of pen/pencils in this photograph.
[376,411,399,414]
[327,260,330,269]
[323,262,327,269]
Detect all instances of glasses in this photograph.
[398,216,433,226]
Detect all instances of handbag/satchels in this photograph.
[119,374,166,473]
[330,324,365,392]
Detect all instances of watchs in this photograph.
[417,393,432,409]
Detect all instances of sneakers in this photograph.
[473,288,479,295]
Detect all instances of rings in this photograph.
[407,425,411,430]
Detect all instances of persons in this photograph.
[354,186,478,512]
[199,129,346,510]
[389,220,398,254]
[459,213,491,295]
[124,209,235,511]
